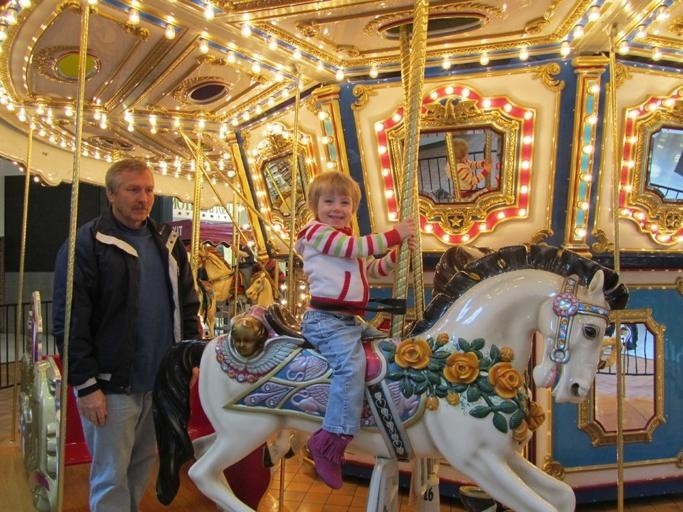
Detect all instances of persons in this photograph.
[290,169,419,492]
[51,158,205,511]
[443,138,494,198]
[229,316,269,357]
[230,229,256,305]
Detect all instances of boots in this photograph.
[305,428,354,489]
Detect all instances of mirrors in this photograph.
[578,309,666,447]
[615,84,683,248]
[251,121,319,228]
[372,84,538,246]
